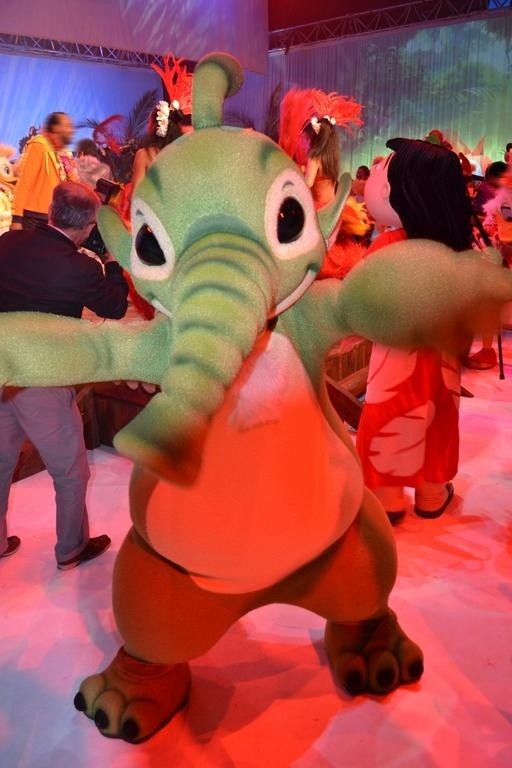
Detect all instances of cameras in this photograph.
[78,179,121,257]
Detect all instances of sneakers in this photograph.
[0,534,22,557]
[55,533,113,570]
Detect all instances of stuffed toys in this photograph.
[0,52,512,745]
